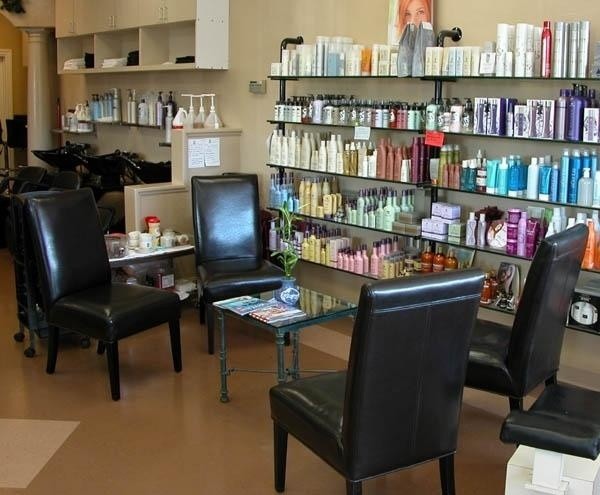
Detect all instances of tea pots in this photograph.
[105,234,126,259]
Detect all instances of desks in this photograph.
[211,282,359,401]
[106,235,197,310]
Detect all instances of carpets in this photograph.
[1,418,80,488]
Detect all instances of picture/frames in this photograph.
[387,0,434,51]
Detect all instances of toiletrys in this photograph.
[129,215,190,254]
[60,87,224,145]
[269,20,600,335]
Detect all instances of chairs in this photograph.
[0,113,27,167]
[465,224,590,416]
[1,165,125,254]
[23,188,185,401]
[190,173,298,354]
[269,267,486,494]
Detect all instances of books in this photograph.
[211,296,308,324]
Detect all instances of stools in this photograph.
[500,378,599,495]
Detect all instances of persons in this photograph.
[394,0,432,41]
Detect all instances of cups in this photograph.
[139,233,152,250]
[126,231,141,249]
[160,228,177,248]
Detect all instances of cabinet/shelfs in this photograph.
[260,64,600,337]
[53,118,172,149]
[53,0,230,75]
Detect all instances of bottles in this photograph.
[150,218,160,247]
[144,216,156,229]
[149,265,165,288]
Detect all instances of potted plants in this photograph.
[268,201,304,306]
